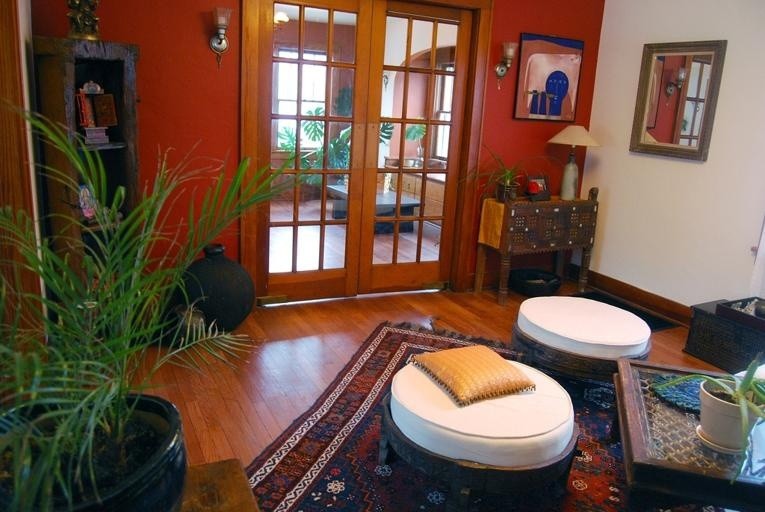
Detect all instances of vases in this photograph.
[175,243,254,332]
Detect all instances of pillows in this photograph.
[406,344,536,407]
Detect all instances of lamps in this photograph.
[274,12,289,31]
[209,7,232,68]
[666,68,686,94]
[547,126,599,201]
[496,40,518,79]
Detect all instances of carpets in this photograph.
[244,320,740,512]
[572,292,680,333]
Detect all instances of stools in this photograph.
[513,295,652,381]
[378,362,578,512]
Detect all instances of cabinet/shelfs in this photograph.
[32,35,139,290]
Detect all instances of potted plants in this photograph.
[0,104,315,512]
[277,108,395,199]
[459,145,528,202]
[653,354,765,487]
[406,117,427,167]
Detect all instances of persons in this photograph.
[81,190,88,205]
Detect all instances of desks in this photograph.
[683,299,765,375]
[473,193,599,305]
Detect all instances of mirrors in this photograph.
[629,38,728,162]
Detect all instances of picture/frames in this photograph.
[646,56,665,129]
[527,175,551,202]
[512,33,585,123]
